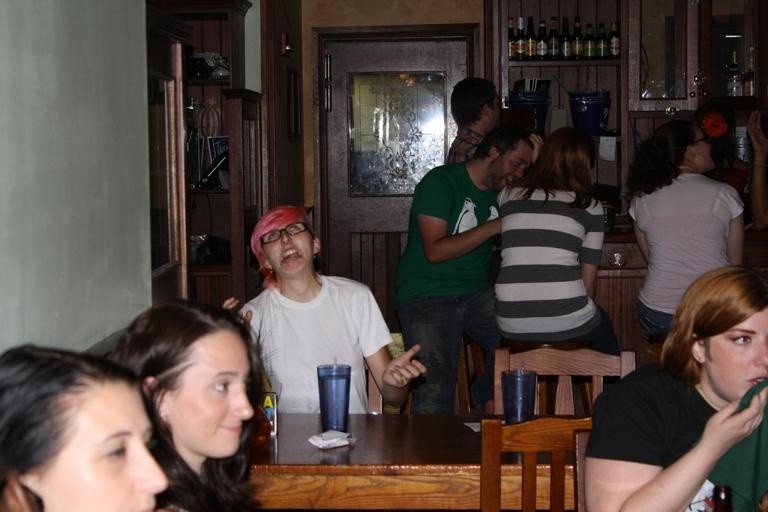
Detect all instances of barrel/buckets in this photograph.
[570,91,613,136]
[726,136,752,191]
[510,91,549,134]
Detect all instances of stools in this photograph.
[457,333,486,413]
[502,339,593,416]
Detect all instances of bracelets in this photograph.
[753,164,766,168]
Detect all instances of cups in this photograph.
[509,91,551,136]
[188,53,232,80]
[568,89,612,135]
[261,391,278,436]
[501,369,536,425]
[607,246,625,268]
[316,364,351,435]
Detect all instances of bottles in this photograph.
[726,49,743,96]
[507,14,620,62]
[744,45,755,97]
[711,484,733,512]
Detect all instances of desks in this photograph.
[239,413,574,510]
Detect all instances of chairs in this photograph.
[494,348,635,415]
[480,417,593,512]
[574,429,593,512]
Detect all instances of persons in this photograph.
[76,296,272,512]
[625,110,745,346]
[493,127,622,385]
[220,205,428,414]
[395,124,547,414]
[747,110,768,233]
[584,265,768,512]
[0,342,171,512]
[444,77,501,164]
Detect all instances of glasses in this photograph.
[681,136,714,154]
[259,221,316,249]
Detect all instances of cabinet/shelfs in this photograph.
[500,0,758,214]
[185,87,264,312]
